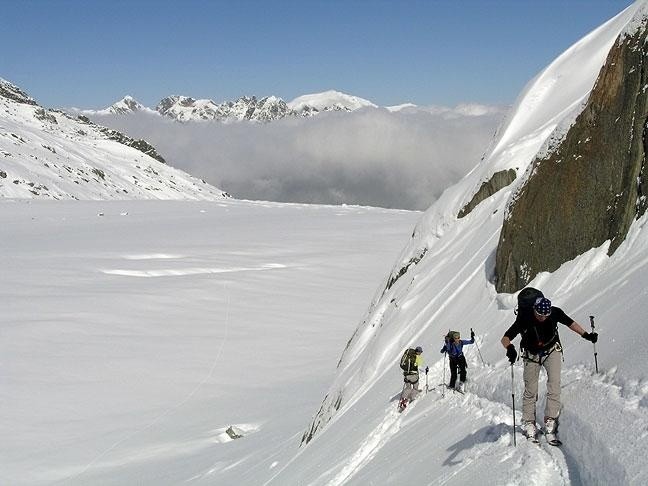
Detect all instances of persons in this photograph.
[399,346,429,410]
[501,297,599,445]
[439,330,475,394]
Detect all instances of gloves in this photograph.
[582,332,598,343]
[506,344,517,364]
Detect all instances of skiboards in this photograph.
[398,388,422,413]
[515,419,561,450]
[444,383,465,396]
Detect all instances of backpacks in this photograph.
[399,348,418,372]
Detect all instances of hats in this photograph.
[416,347,423,352]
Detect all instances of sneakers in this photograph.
[525,428,537,441]
[544,425,558,442]
